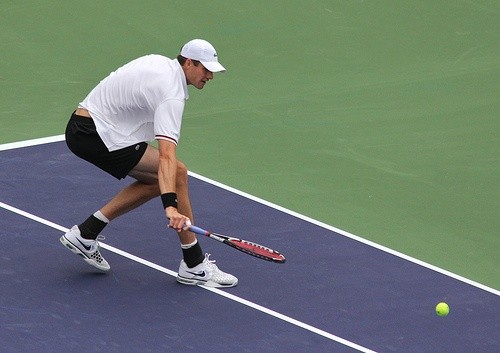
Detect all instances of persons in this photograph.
[59,40,239,289]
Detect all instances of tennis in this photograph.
[436,302,450,317]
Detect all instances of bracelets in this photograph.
[162,192,178,210]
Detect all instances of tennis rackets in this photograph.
[167,218,287,265]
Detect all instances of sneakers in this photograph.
[60,225,110,272]
[177,253,239,287]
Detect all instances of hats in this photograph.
[181,39,226,72]
